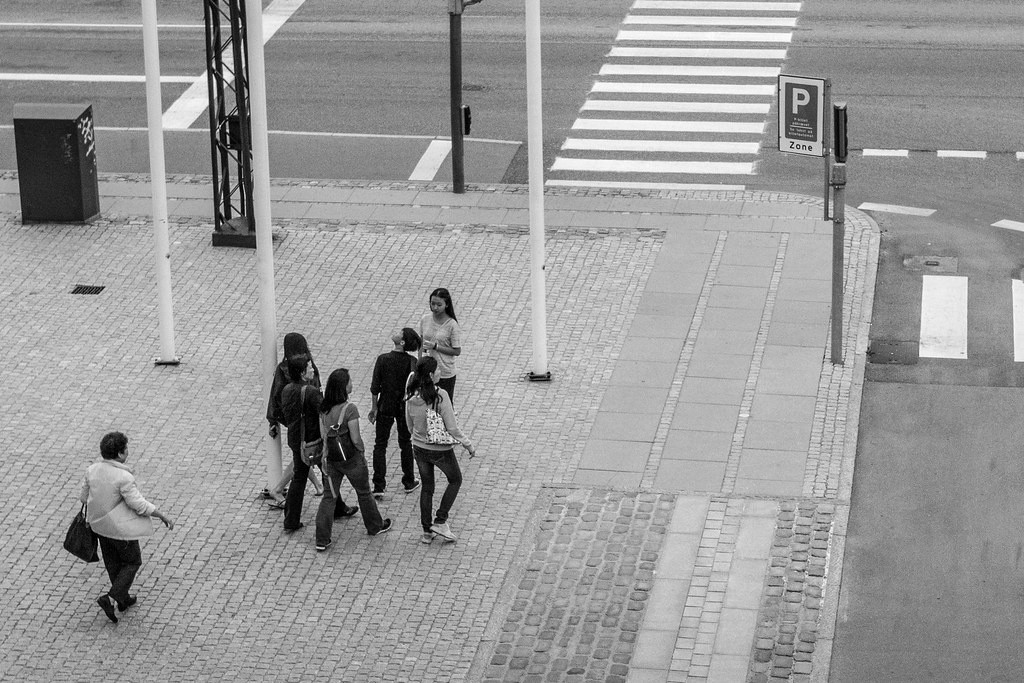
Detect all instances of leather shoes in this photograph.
[97,594,118,623]
[118,596,137,612]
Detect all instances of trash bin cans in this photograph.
[11,101,101,225]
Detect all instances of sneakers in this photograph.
[430,521,456,539]
[376,518,392,535]
[422,532,437,544]
[316,539,332,550]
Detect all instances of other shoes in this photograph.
[371,489,384,497]
[283,523,303,531]
[344,506,358,516]
[404,480,420,493]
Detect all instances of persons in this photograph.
[280,354,359,531]
[367,327,422,497]
[406,356,475,543]
[418,287,461,411]
[315,368,392,550]
[265,333,324,509]
[78,431,174,625]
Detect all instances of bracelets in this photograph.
[160,512,164,520]
[433,343,438,351]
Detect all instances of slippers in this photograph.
[268,500,286,509]
[315,489,324,496]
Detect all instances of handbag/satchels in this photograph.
[301,438,324,467]
[426,385,462,445]
[327,402,355,462]
[63,503,99,563]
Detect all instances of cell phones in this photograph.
[271,425,279,439]
